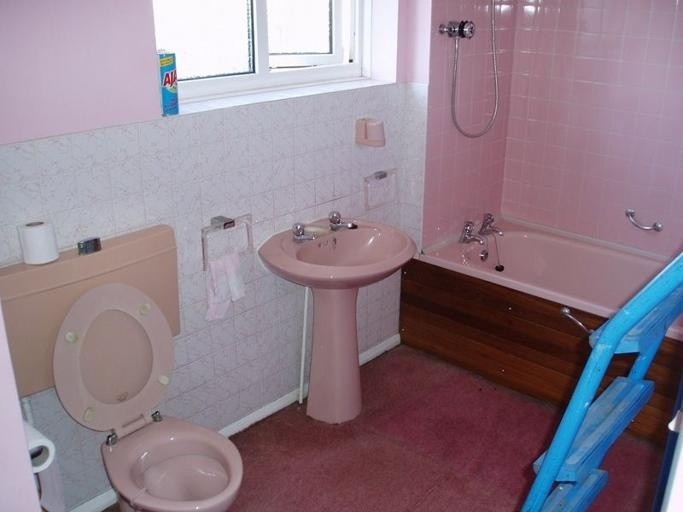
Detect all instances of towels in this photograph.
[205,252,246,320]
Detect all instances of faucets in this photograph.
[458,220,485,245]
[291,222,320,243]
[328,211,358,232]
[477,212,504,237]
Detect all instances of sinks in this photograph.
[256,217,418,289]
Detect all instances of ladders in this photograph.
[519,251,683,512]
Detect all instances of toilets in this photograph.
[0,222,245,512]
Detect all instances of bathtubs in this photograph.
[422,211,682,344]
